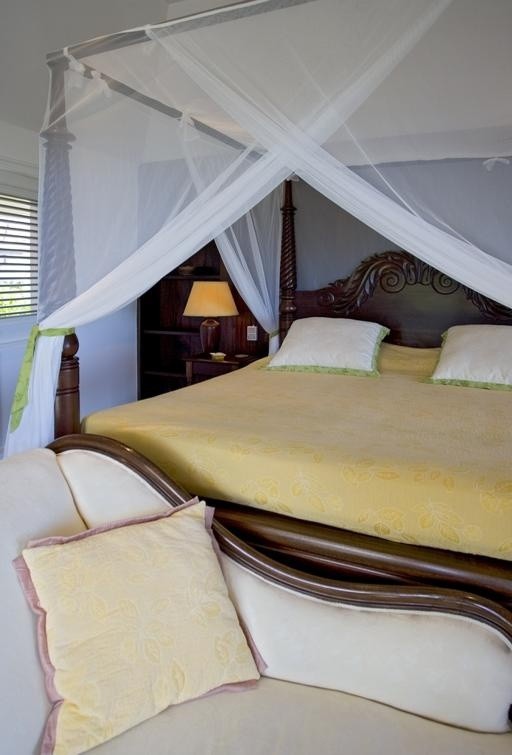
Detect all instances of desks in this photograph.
[181,351,254,387]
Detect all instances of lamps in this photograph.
[181,280,239,355]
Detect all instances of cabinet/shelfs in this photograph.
[136,158,266,402]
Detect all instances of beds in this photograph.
[37,1,512,605]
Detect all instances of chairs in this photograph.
[2,434,512,755]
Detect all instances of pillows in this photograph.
[262,313,512,395]
[12,490,269,755]
